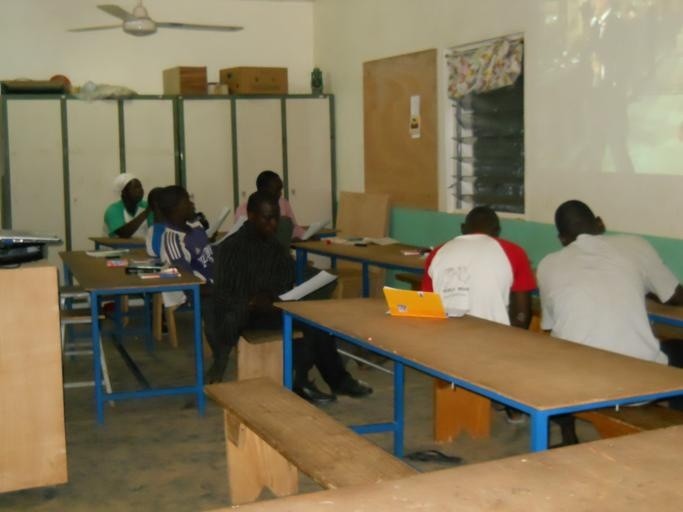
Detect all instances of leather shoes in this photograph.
[294,381,336,402]
[335,376,373,398]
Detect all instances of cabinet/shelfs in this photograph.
[0,259,69,492]
[2,92,339,310]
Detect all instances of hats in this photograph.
[116,173,134,190]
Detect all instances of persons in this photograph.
[537,200,683,448]
[420,206,537,425]
[200,191,373,404]
[99,170,303,272]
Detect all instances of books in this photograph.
[86,249,182,279]
[382,286,449,319]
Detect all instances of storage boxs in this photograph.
[163,64,208,95]
[218,66,288,96]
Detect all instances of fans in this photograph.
[68,0,243,35]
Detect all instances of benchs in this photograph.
[202,376,421,503]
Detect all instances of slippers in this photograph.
[406,449,462,467]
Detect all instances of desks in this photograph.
[273,234,683,459]
[55,230,211,426]
[193,425,683,512]
